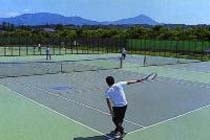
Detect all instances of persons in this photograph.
[38,43,41,52]
[46,46,51,60]
[120,47,126,60]
[104,75,144,138]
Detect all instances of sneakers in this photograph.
[111,131,119,136]
[121,130,127,138]
